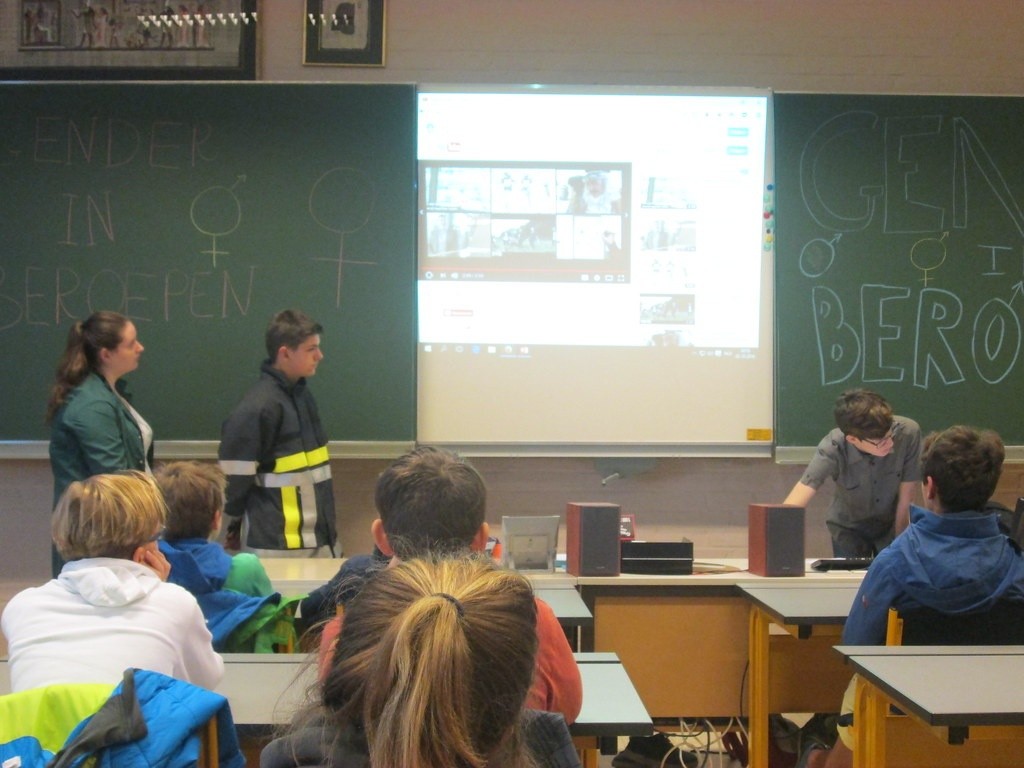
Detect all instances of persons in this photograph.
[217,309,344,616]
[260,521,582,768]
[782,385,922,558]
[566,171,621,215]
[794,423,1024,768]
[316,443,583,726]
[299,544,392,633]
[152,458,302,735]
[0,469,224,691]
[39,311,154,579]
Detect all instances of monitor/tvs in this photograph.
[500,516,560,573]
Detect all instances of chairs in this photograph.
[0,670,247,768]
[836,601,1024,737]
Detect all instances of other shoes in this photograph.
[794,729,831,768]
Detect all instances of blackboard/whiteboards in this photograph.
[0,79,416,460]
[769,90,1024,466]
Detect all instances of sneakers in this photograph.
[611,733,699,768]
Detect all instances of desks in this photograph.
[0,554,1024,768]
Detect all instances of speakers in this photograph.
[748,503,807,579]
[565,502,621,578]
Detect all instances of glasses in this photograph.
[853,419,903,450]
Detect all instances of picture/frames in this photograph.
[301,0,386,67]
[0,0,263,81]
